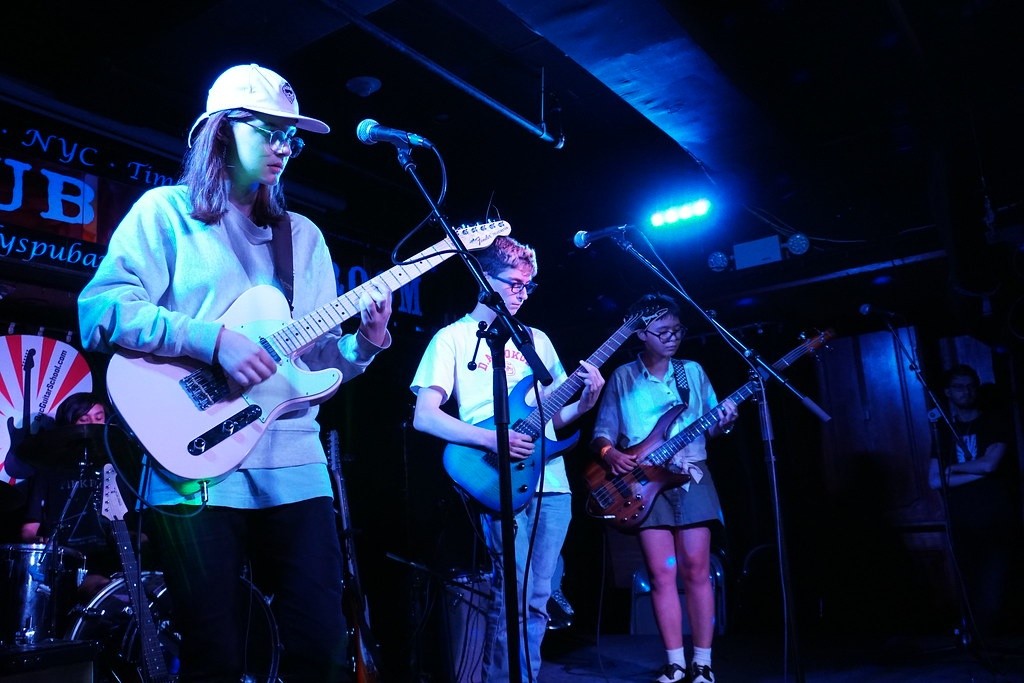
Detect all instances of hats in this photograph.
[188,64,331,149]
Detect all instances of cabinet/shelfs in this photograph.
[812,326,971,654]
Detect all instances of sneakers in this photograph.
[690,663,715,683]
[654,664,690,683]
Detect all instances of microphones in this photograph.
[573,224,636,248]
[860,305,894,317]
[357,118,435,150]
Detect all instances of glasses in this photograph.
[489,273,538,294]
[646,325,687,344]
[241,118,305,158]
[951,382,973,392]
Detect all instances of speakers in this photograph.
[437,569,493,683]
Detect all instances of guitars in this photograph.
[100,459,182,683]
[105,219,513,494]
[581,327,837,526]
[441,304,669,514]
[323,428,384,683]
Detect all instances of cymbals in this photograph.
[14,423,133,473]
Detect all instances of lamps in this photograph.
[707,234,810,273]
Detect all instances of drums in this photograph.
[37,466,130,548]
[0,544,107,667]
[66,565,279,683]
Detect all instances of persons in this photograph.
[592,294,738,683]
[78,63,392,683]
[928,364,1010,645]
[20,394,180,683]
[410,236,605,683]
